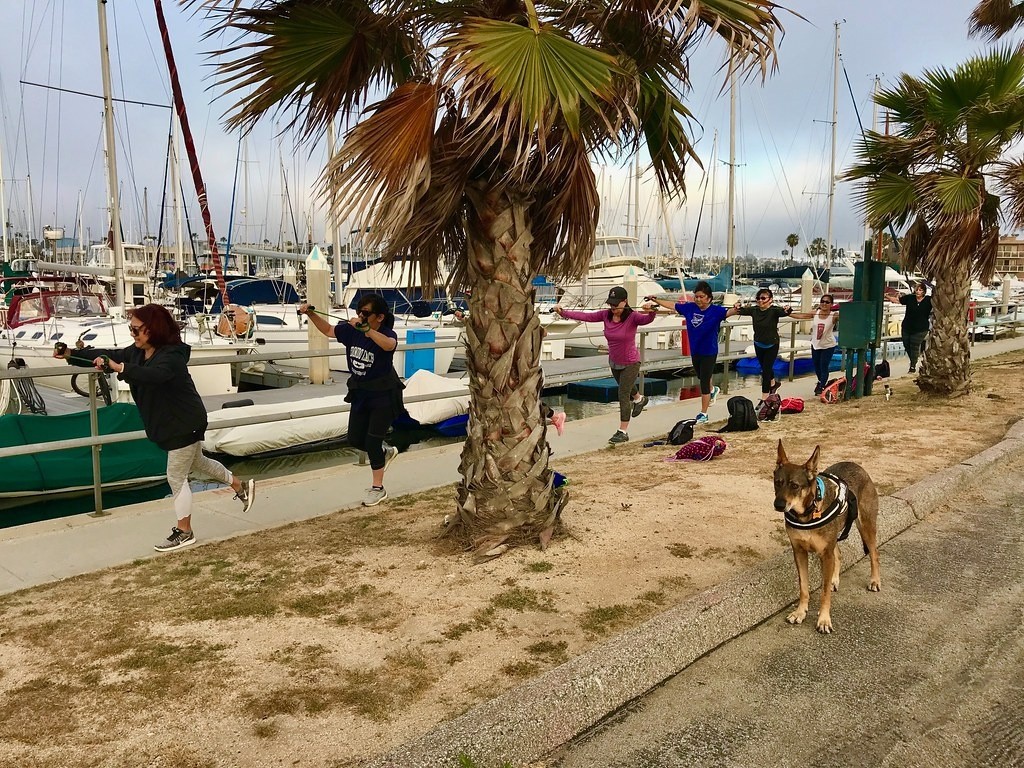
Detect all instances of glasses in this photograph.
[821,300,832,304]
[356,309,378,318]
[756,296,771,300]
[609,303,619,307]
[128,323,145,336]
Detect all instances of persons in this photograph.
[889,284,932,373]
[52,306,256,553]
[300,295,406,505]
[551,287,659,445]
[731,288,840,406]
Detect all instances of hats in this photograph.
[605,286,627,305]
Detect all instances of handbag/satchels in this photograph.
[820,359,890,404]
[662,435,726,463]
[780,397,804,414]
[659,419,697,445]
[757,394,782,423]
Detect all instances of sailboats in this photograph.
[0,0,1023,506]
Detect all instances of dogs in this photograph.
[773,437,880,633]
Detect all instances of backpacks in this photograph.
[706,396,760,433]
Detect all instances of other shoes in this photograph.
[908,366,916,374]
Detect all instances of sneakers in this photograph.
[154,526,196,552]
[708,386,720,407]
[755,399,766,413]
[769,381,782,395]
[608,430,629,444]
[233,479,255,513]
[551,412,566,436]
[632,396,649,417]
[383,446,398,472]
[813,382,826,394]
[694,412,709,425]
[362,487,387,507]
